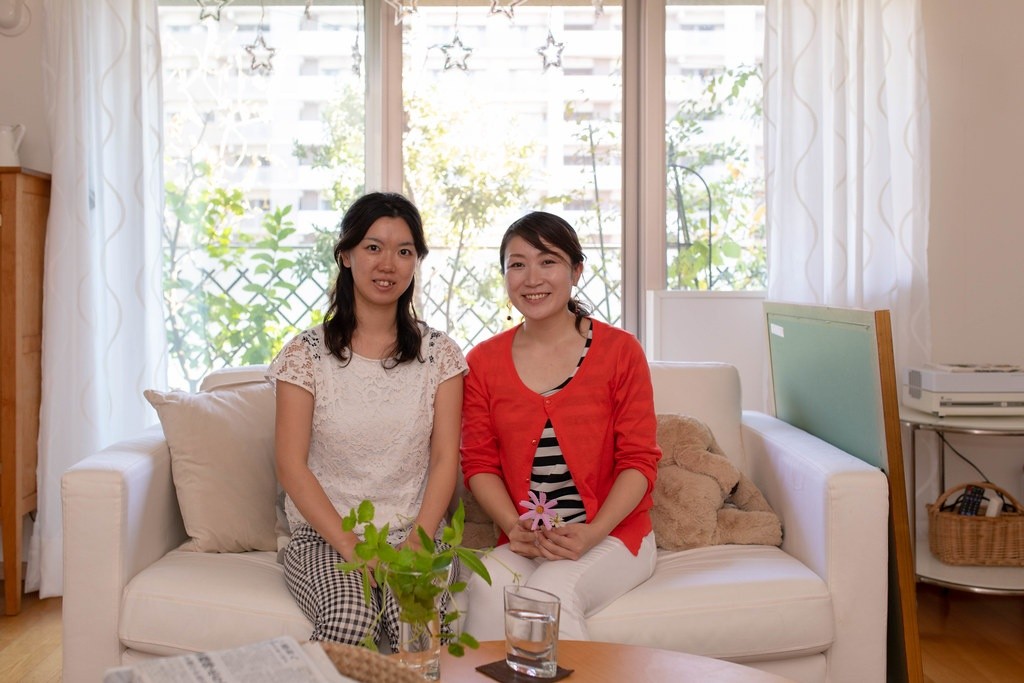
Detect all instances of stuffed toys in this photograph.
[460,414,783,551]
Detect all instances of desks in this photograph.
[387,640,793,683]
[899,405,1024,601]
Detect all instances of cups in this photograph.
[503,585,561,678]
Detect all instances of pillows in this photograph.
[144,382,280,553]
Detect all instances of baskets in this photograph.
[926,482,1024,567]
[297,640,431,683]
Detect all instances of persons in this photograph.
[458,212,657,643]
[264,193,469,654]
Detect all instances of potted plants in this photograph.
[61,361,889,683]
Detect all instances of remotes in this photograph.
[958,484,985,516]
[986,497,1004,517]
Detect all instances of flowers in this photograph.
[518,491,564,533]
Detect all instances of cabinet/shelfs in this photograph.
[0,166,57,616]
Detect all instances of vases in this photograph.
[385,571,440,680]
